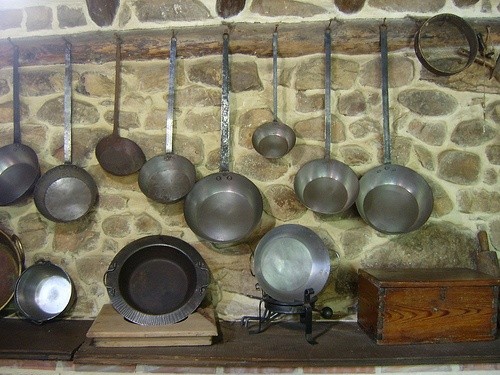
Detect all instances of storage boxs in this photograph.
[357,268,500,345]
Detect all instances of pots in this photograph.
[0,228,25,311]
[249,224,341,303]
[103,234,211,326]
[14,257,76,326]
